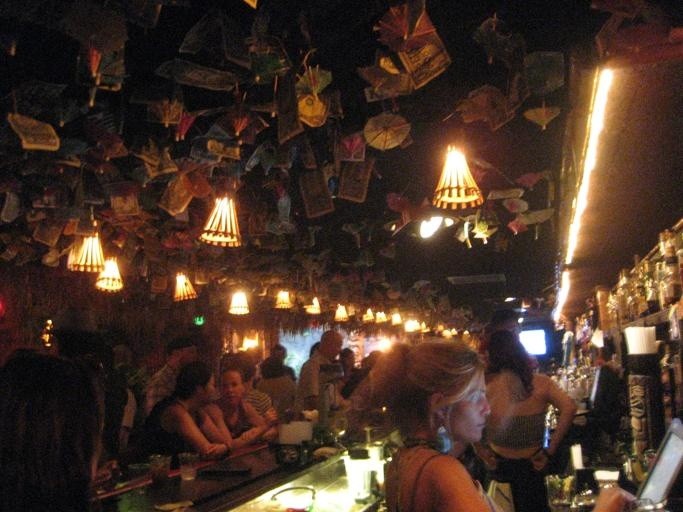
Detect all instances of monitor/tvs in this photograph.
[518,324,554,361]
[633,419,683,504]
[587,363,617,410]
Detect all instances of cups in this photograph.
[273,484,315,511]
[128,452,200,494]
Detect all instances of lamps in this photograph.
[195,193,245,250]
[73,222,125,292]
[172,271,199,303]
[276,289,292,310]
[431,134,484,211]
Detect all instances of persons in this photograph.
[50,310,632,486]
[464,323,579,511]
[359,339,639,511]
[0,349,107,512]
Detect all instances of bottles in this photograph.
[603,229,681,323]
